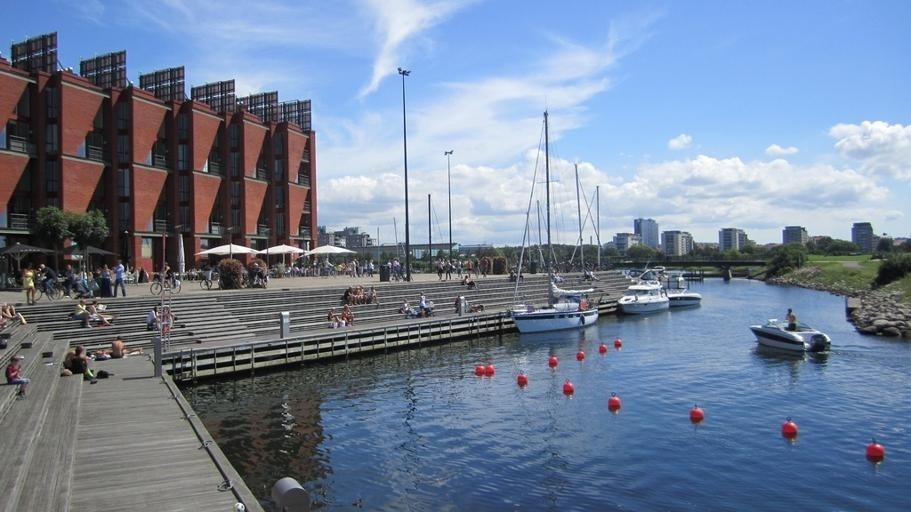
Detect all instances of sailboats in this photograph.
[503,112,602,333]
[510,162,611,273]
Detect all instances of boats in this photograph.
[749,318,835,354]
[617,265,703,314]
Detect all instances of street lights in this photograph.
[227,225,233,259]
[263,227,271,268]
[123,228,129,268]
[299,229,306,266]
[443,148,456,271]
[394,65,415,281]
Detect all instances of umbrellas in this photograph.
[0,240,56,269]
[61,242,115,270]
[194,244,261,258]
[259,245,308,263]
[301,242,356,264]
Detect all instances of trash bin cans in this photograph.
[380,265,390,282]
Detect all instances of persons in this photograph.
[2,262,144,328]
[147,303,172,331]
[786,308,796,331]
[245,259,374,288]
[6,355,31,395]
[454,294,481,314]
[393,257,404,280]
[328,285,376,329]
[579,294,594,310]
[66,335,124,380]
[495,257,617,282]
[400,292,434,318]
[165,262,217,289]
[437,256,488,289]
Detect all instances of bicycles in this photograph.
[150,271,183,295]
[23,274,66,301]
[197,273,212,290]
